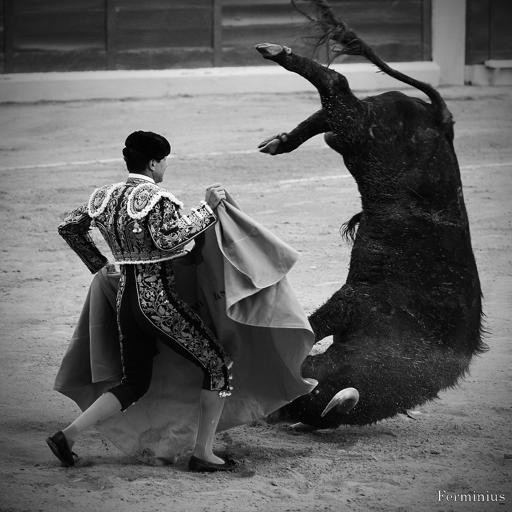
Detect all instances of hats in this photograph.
[123,131,170,168]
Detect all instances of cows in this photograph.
[259,0,488,432]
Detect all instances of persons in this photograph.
[44,130,238,472]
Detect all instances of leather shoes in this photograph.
[189,456,239,472]
[45,431,74,466]
